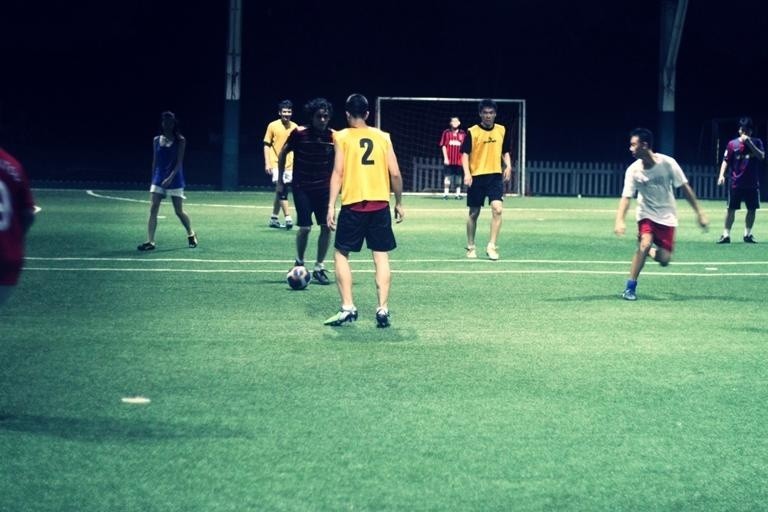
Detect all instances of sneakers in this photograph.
[486,244,498,260]
[744,235,758,243]
[622,289,637,300]
[188,233,197,248]
[270,216,393,326]
[717,235,730,244]
[466,246,476,257]
[137,241,155,251]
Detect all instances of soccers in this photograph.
[287,266,311,290]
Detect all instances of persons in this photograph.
[263,99,299,231]
[274,96,339,286]
[614,127,708,301]
[138,109,198,252]
[1,148,34,326]
[438,116,467,202]
[323,91,405,329]
[459,100,511,261]
[716,114,765,248]
